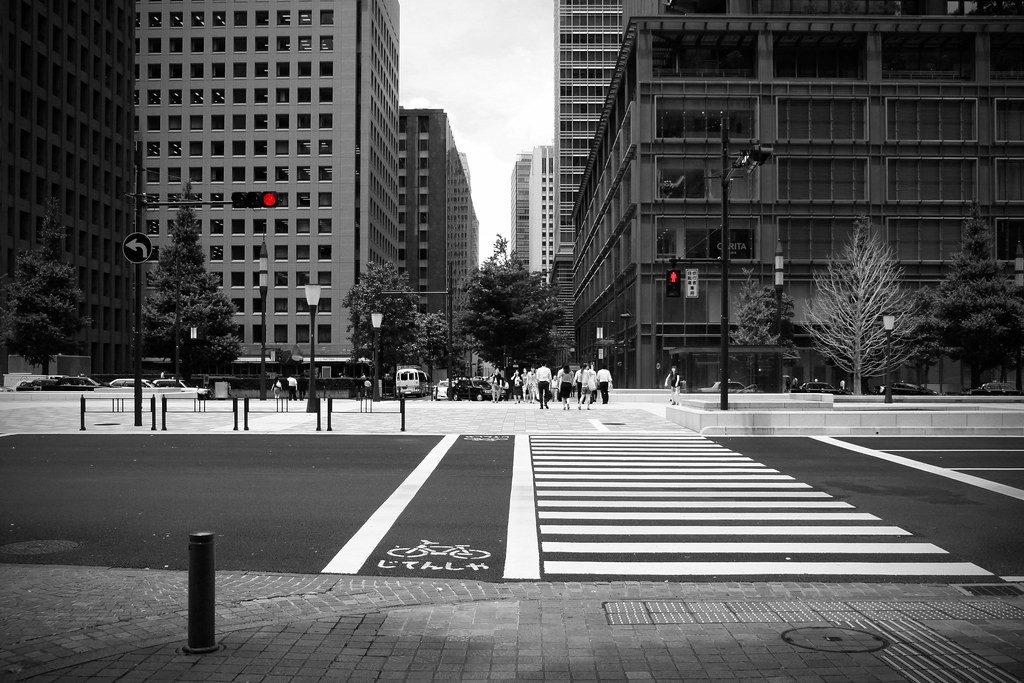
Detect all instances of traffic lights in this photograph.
[232,191,289,208]
[666,270,682,297]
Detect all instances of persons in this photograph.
[490,361,599,404]
[783,375,798,393]
[557,363,575,410]
[536,361,552,409]
[595,364,612,404]
[840,377,849,395]
[297,373,309,401]
[665,365,681,405]
[363,378,372,398]
[286,374,298,401]
[270,376,282,399]
[578,363,596,410]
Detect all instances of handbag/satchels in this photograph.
[588,377,596,391]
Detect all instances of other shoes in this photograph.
[677,402,679,405]
[540,407,543,409]
[533,402,535,404]
[514,402,517,404]
[491,400,495,403]
[518,401,520,403]
[526,401,528,403]
[530,401,532,403]
[544,405,549,409]
[523,401,525,402]
[496,401,498,403]
[563,407,565,410]
[578,407,581,410]
[587,407,590,410]
[670,400,672,401]
[567,406,569,410]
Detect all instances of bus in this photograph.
[396,369,434,398]
[228,356,374,378]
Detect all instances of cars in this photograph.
[960,380,1022,395]
[433,376,507,401]
[697,381,764,393]
[152,378,210,399]
[873,381,938,395]
[110,379,155,389]
[16,376,109,392]
[788,382,853,395]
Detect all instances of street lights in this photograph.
[305,284,322,413]
[882,315,895,403]
[371,312,383,402]
